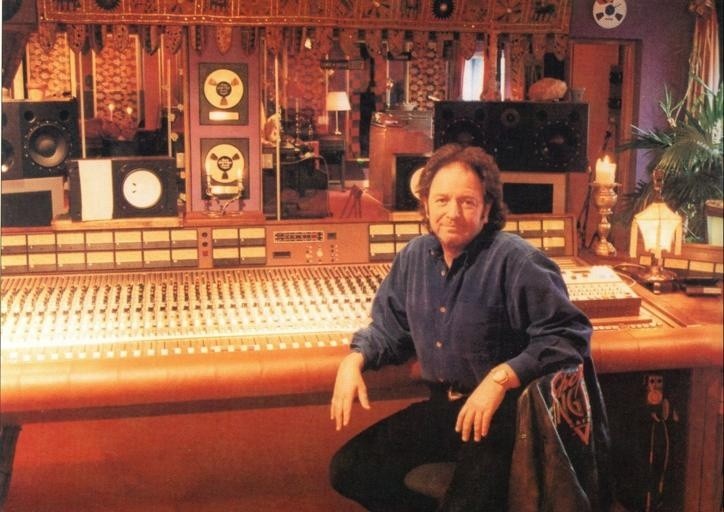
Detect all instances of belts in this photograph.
[435,386,464,403]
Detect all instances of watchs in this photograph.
[490,366,512,388]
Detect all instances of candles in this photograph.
[236,169,240,179]
[596,155,616,183]
[205,164,211,175]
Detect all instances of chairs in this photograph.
[402,357,611,510]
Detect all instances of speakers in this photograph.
[67,158,177,220]
[434,100,588,172]
[20,101,83,179]
[1,102,23,180]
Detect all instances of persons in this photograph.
[328,142,595,512]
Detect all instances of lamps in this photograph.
[631,169,683,295]
[325,90,351,135]
